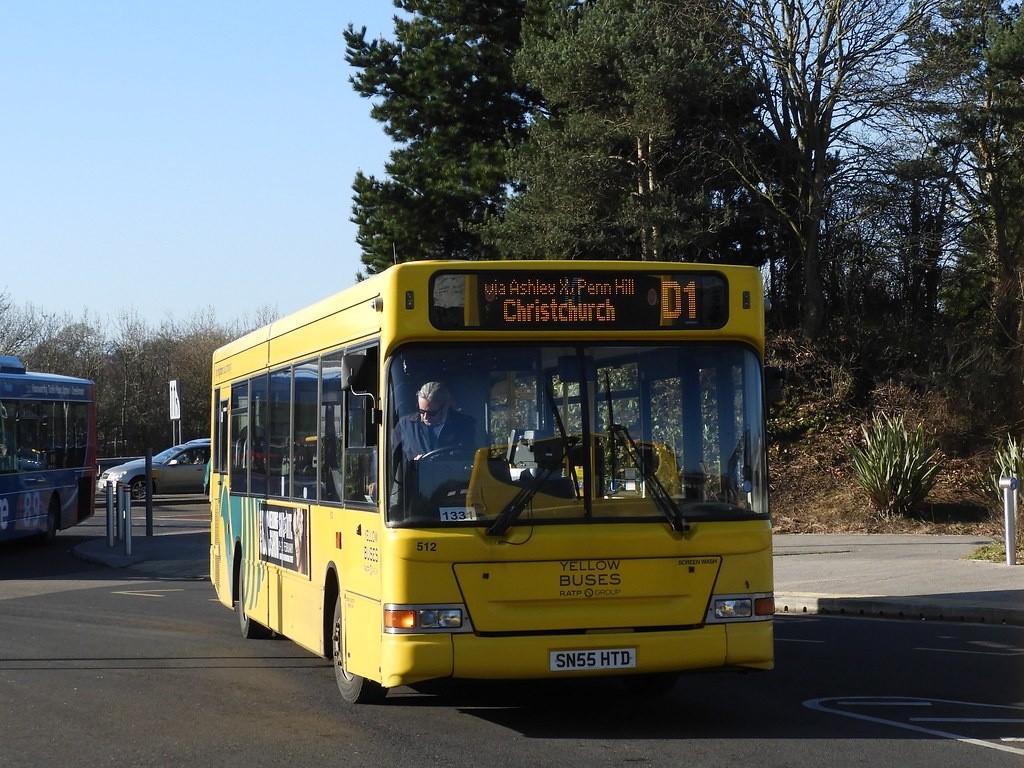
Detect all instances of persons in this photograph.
[367,382,480,508]
[183,452,204,465]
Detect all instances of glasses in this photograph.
[416,403,446,416]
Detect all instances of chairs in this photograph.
[229,438,332,501]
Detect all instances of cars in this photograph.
[122,438,211,465]
[98,443,212,500]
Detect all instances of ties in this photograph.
[429,423,442,452]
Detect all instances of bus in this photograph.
[208,260,771,703]
[0,354,97,546]
[232,368,330,499]
